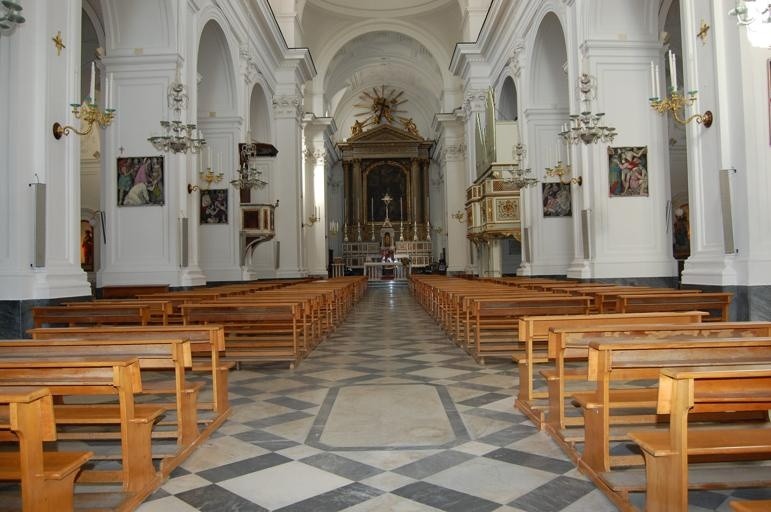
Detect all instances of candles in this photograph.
[343,199,347,223]
[548,142,569,169]
[312,204,319,219]
[88,60,112,114]
[329,219,338,232]
[650,49,677,97]
[357,198,359,220]
[399,197,402,218]
[370,197,373,219]
[426,196,429,218]
[199,146,223,175]
[413,196,416,217]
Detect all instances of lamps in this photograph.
[229,131,267,191]
[505,143,540,188]
[147,77,206,154]
[556,74,617,145]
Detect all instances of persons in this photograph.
[544,184,571,216]
[610,147,648,197]
[202,191,226,223]
[119,158,165,206]
